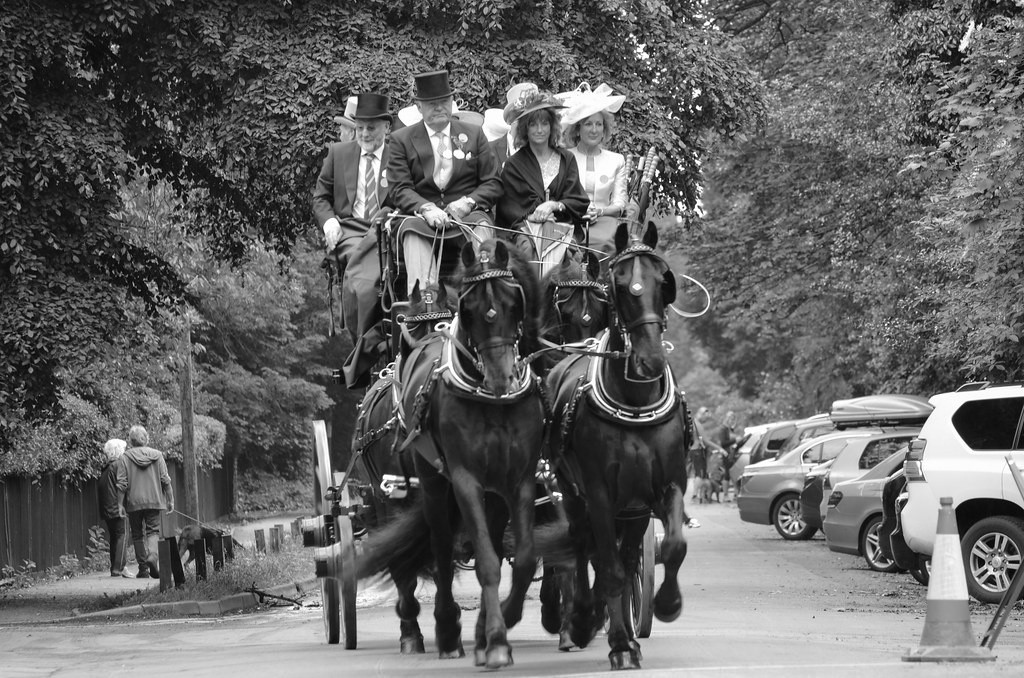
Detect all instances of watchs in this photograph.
[557,200,564,212]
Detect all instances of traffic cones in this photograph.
[903,497,991,665]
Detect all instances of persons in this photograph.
[312,69,628,305]
[97,424,174,579]
[688,406,742,505]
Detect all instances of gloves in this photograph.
[419,201,453,231]
[443,195,475,218]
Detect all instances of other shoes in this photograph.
[136,571,149,578]
[111,567,135,579]
[146,558,160,579]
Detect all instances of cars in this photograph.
[822,448,926,584]
[733,392,937,537]
[897,382,1024,600]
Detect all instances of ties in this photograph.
[364,152,379,223]
[436,132,448,158]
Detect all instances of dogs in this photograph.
[179,525,245,573]
[693,451,723,504]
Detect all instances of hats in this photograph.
[397,99,484,129]
[554,84,626,134]
[350,93,394,124]
[334,96,358,129]
[507,90,570,125]
[412,70,457,100]
[480,108,510,140]
[503,83,539,121]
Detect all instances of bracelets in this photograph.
[598,207,603,216]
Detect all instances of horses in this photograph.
[354,220,688,666]
